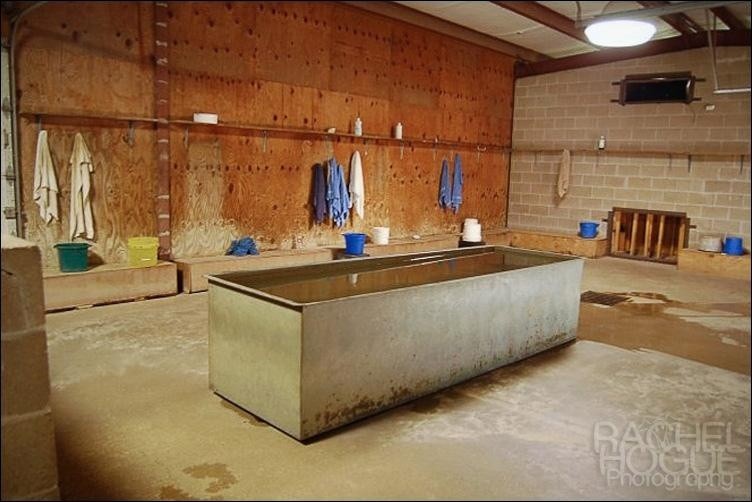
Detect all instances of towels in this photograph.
[334,165,352,228]
[325,158,341,220]
[438,160,452,210]
[345,150,366,220]
[33,130,62,225]
[69,132,98,241]
[556,149,571,198]
[312,162,327,222]
[453,153,464,212]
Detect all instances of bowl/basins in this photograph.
[191,112,219,125]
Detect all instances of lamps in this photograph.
[584,21,655,48]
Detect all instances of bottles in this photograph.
[395,122,402,139]
[598,136,605,150]
[355,118,362,135]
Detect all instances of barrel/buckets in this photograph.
[53,243,92,272]
[341,232,365,254]
[128,236,159,268]
[373,227,390,245]
[723,236,743,255]
[580,222,597,238]
